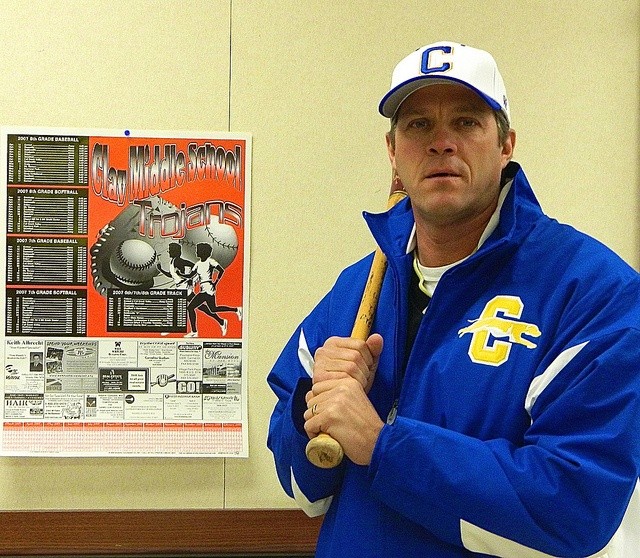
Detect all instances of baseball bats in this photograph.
[305,167,408,470]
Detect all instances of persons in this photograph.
[156,243,228,336]
[176,243,243,338]
[30,354,43,371]
[266,41,640,557]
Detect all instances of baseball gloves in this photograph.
[90,194,201,300]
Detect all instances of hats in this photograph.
[379,40,511,126]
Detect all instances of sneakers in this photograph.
[234,306,242,320]
[219,318,228,336]
[184,330,198,337]
[160,331,170,335]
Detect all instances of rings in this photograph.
[312,404,318,415]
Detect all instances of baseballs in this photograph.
[177,214,239,271]
[110,239,162,287]
